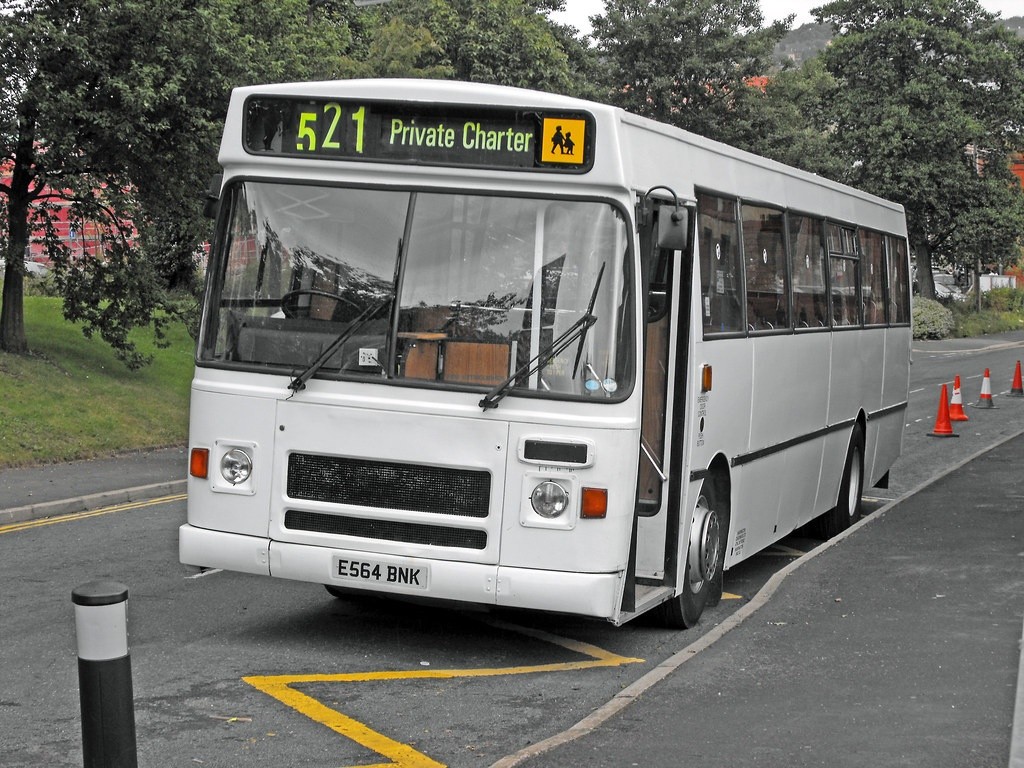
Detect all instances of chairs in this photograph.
[748,297,777,325]
[797,288,898,327]
[330,272,412,355]
[709,295,742,327]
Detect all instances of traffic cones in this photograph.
[1007,360,1024,397]
[971,368,1000,409]
[927,384,960,438]
[948,375,969,421]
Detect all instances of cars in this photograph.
[0,259,52,279]
[931,267,963,298]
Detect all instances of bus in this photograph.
[176,74,913,631]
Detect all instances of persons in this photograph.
[332,280,411,348]
